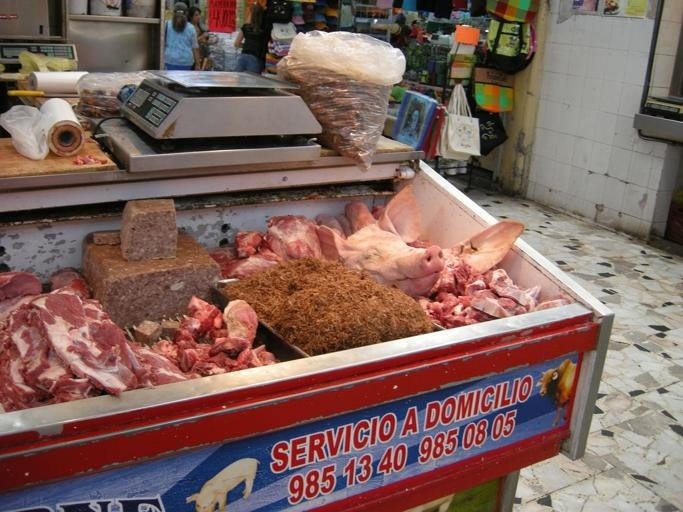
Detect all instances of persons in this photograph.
[164,2,200,70]
[234,4,268,75]
[188,10,208,56]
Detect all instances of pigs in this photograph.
[184,456,262,512]
[315,184,448,298]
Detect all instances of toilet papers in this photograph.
[40,98,84,156]
[26,70,90,93]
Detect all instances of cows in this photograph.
[534,357,578,428]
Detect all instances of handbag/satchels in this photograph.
[486,19,537,75]
[485,0,539,23]
[390,89,444,162]
[436,112,509,161]
[474,66,514,113]
[265,0,296,71]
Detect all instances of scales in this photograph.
[0,34,79,65]
[119,69,325,153]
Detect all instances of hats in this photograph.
[292,0,339,32]
